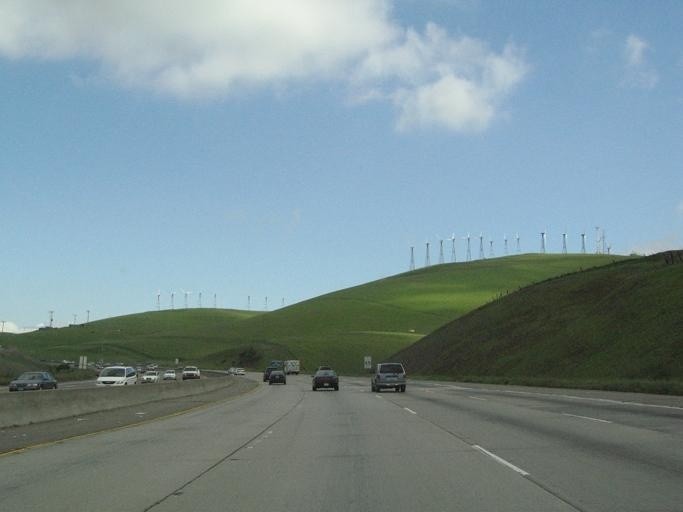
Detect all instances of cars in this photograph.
[227,366,246,376]
[263,359,300,385]
[311,365,338,391]
[182,366,201,381]
[96,362,161,387]
[371,363,406,393]
[9,371,58,392]
[163,369,177,380]
